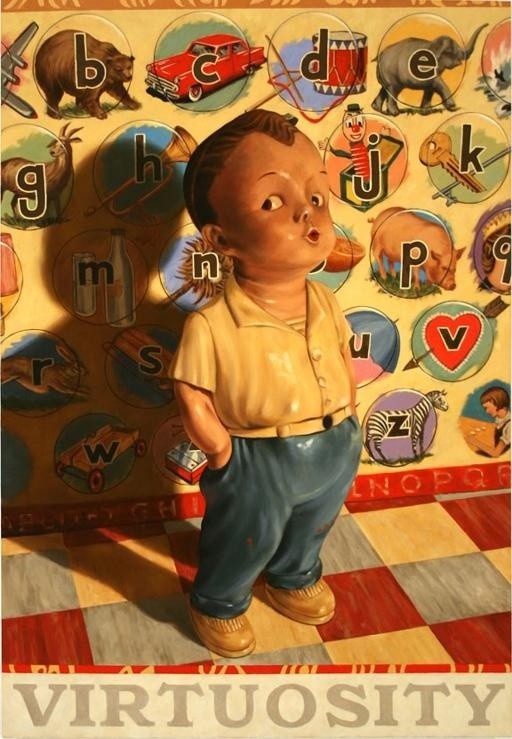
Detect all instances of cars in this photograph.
[142,31,272,106]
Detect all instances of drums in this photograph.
[311,31,367,96]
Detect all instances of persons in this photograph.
[164,109,362,659]
[462,385,510,458]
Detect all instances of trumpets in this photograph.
[86,126,199,221]
